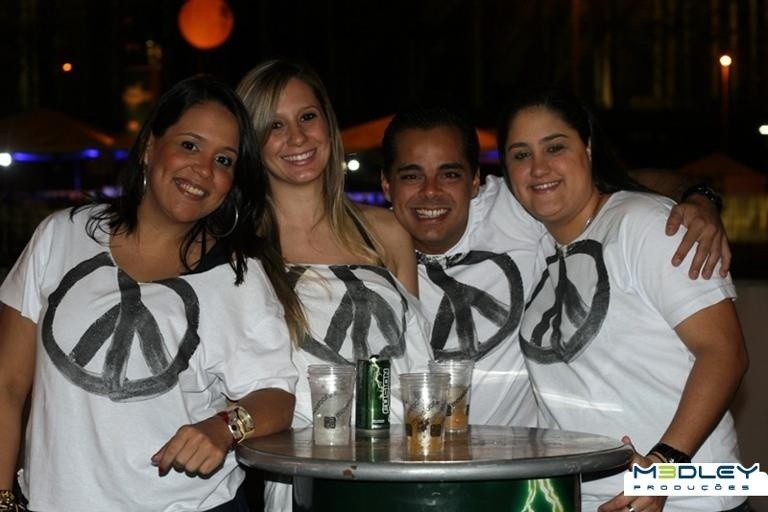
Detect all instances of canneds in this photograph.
[354,440,390,461]
[356,355,390,440]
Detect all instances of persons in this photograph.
[0,56,756,512]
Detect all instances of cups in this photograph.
[399,357,475,456]
[308,364,358,447]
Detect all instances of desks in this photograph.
[235,422,639,511]
